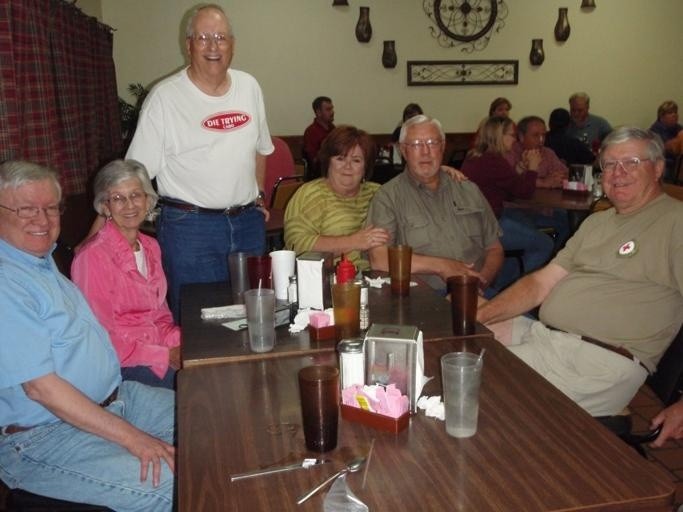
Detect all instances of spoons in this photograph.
[293,456,367,506]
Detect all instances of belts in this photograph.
[0,422,43,439]
[99,385,120,408]
[543,324,651,373]
[161,198,256,214]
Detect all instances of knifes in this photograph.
[226,458,333,483]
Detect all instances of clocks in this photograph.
[420,1,509,56]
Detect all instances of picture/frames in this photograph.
[406,59,519,87]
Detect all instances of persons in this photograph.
[285,127,470,270]
[544,108,595,163]
[262,135,302,234]
[479,126,683,450]
[558,94,611,162]
[76,3,274,328]
[462,114,556,273]
[507,116,578,256]
[366,114,501,309]
[69,159,183,390]
[648,101,683,164]
[1,159,179,512]
[303,97,340,164]
[394,104,427,147]
[471,98,521,148]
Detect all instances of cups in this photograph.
[270,250,296,301]
[446,275,480,337]
[387,244,412,297]
[246,255,273,287]
[297,364,340,454]
[440,352,482,439]
[243,288,275,353]
[229,253,247,297]
[330,283,361,344]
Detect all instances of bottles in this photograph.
[353,278,370,306]
[359,304,369,330]
[331,253,361,341]
[286,275,297,303]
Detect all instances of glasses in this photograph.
[187,32,229,43]
[111,190,144,204]
[598,157,653,171]
[403,138,443,149]
[0,203,63,218]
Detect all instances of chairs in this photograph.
[587,334,680,460]
[260,175,306,253]
